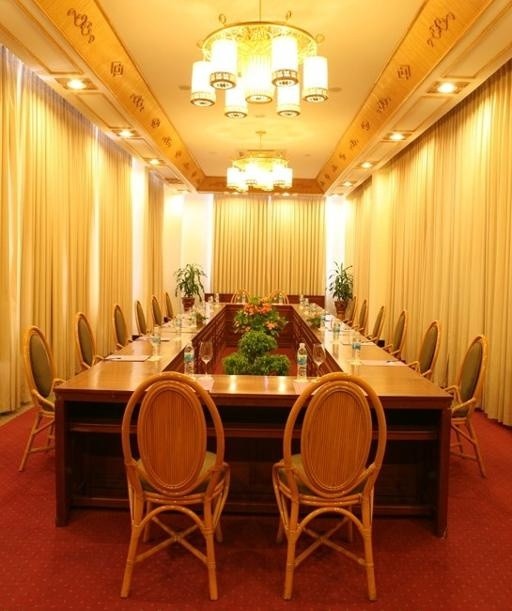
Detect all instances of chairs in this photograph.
[271,373,389,600]
[231,289,250,303]
[342,296,488,479]
[18,289,177,472]
[121,371,231,601]
[269,289,290,304]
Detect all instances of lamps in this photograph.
[190,0,328,119]
[226,131,293,192]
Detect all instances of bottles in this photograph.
[182,340,195,377]
[296,342,308,382]
[151,290,362,364]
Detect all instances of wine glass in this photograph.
[198,341,214,380]
[311,342,328,383]
[148,331,161,361]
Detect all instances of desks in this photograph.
[54,302,454,539]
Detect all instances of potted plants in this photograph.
[327,261,353,318]
[174,263,207,312]
